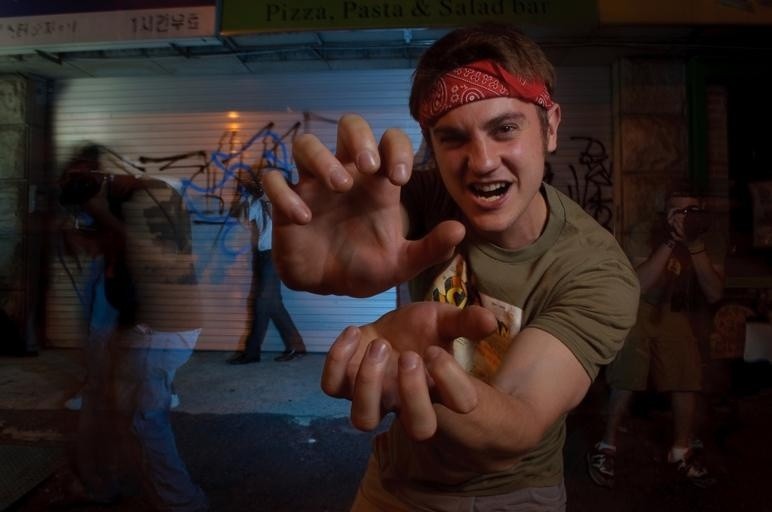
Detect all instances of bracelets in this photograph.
[664,239,675,249]
[687,241,708,256]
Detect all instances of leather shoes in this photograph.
[225,351,248,364]
[274,348,306,361]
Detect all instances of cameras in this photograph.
[680,205,710,238]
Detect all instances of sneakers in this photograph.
[667,443,708,478]
[587,440,616,489]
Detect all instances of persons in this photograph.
[262,24,643,512]
[225,162,309,365]
[58,166,216,512]
[58,138,126,418]
[581,177,729,486]
